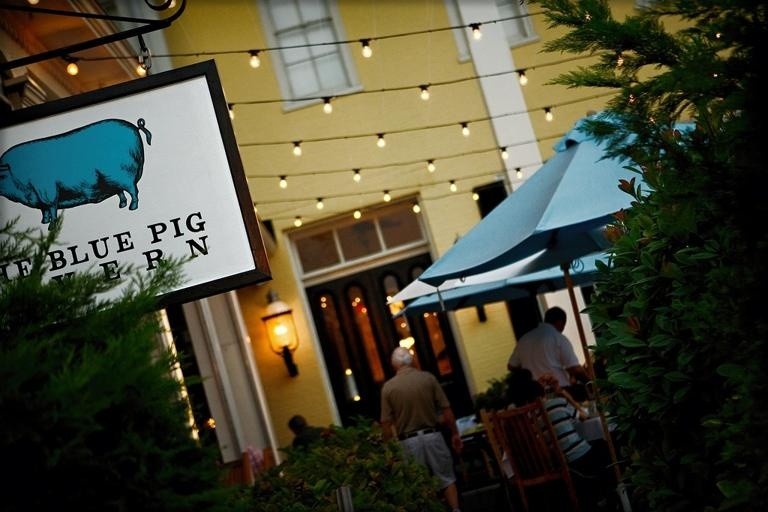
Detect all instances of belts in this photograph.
[397,428,440,441]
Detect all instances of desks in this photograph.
[575,415,633,441]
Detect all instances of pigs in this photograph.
[0,119,152,231]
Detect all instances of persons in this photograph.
[287,415,323,448]
[501,306,613,476]
[380,346,464,512]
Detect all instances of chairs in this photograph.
[480,397,579,512]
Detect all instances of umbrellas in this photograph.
[384,222,638,512]
[391,251,616,319]
[418,108,700,284]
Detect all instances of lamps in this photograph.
[260,288,300,376]
